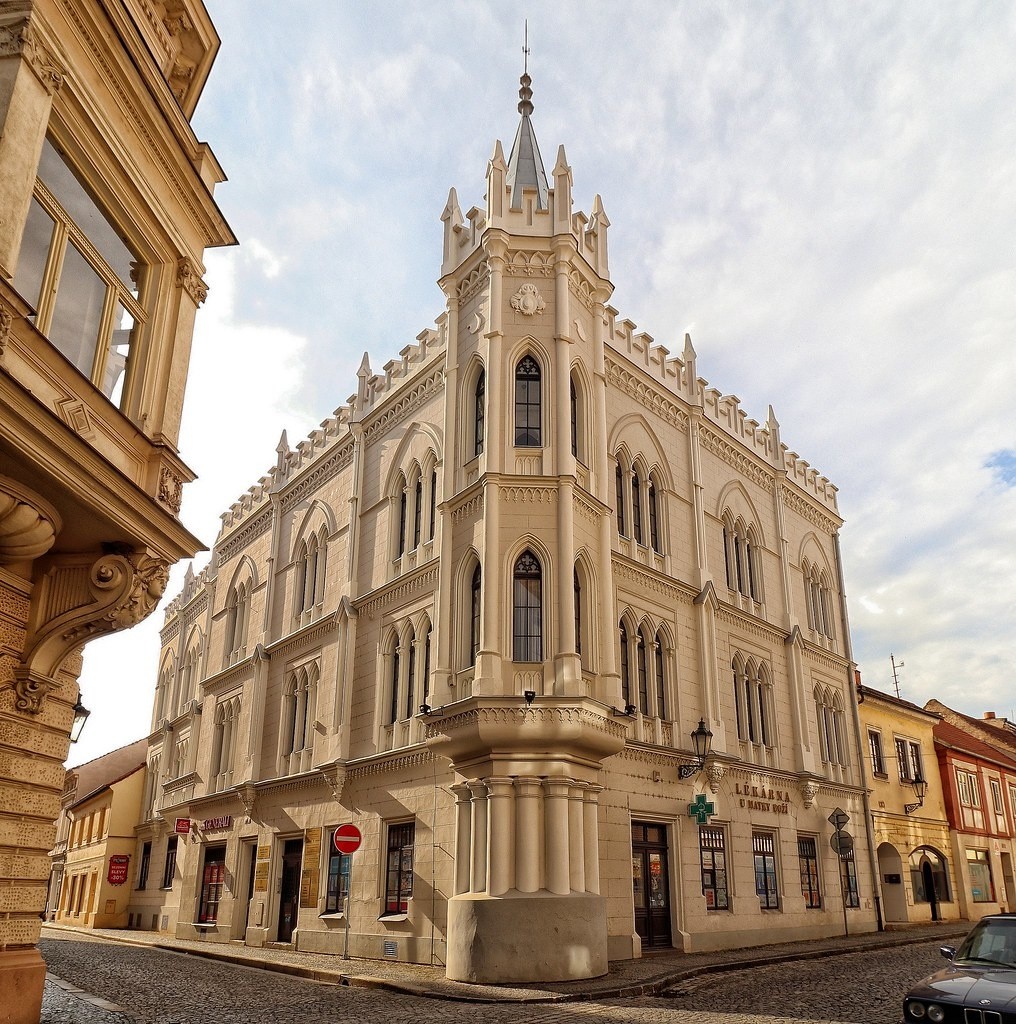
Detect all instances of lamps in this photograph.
[418,704,444,717]
[524,690,534,706]
[613,705,637,717]
[903,773,928,814]
[68,692,90,745]
[677,717,714,780]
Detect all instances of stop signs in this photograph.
[334,824,362,853]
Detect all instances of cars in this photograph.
[902,914,1016,1023]
